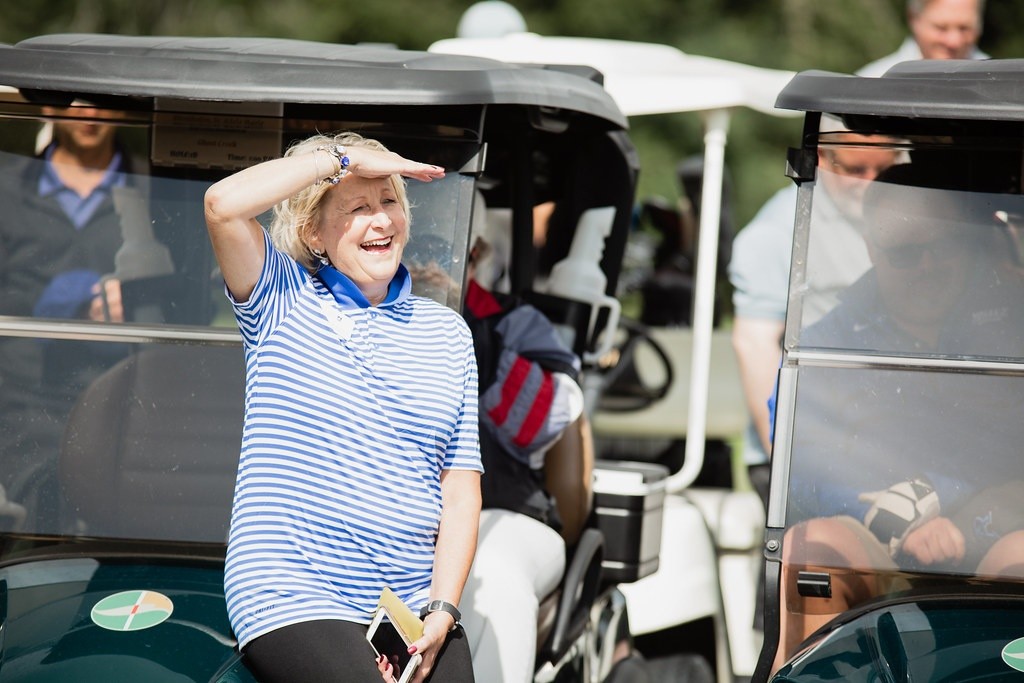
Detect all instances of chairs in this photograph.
[531,410,606,660]
[57,346,246,542]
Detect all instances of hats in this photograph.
[400,173,493,274]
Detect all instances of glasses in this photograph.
[828,155,886,177]
[403,244,462,274]
[872,237,963,268]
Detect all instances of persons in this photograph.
[0,95,213,526]
[396,170,597,683]
[769,161,1024,664]
[201,132,485,683]
[727,107,911,458]
[857,0,992,79]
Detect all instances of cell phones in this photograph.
[365,605,422,683]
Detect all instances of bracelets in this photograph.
[419,600,461,631]
[316,143,350,185]
